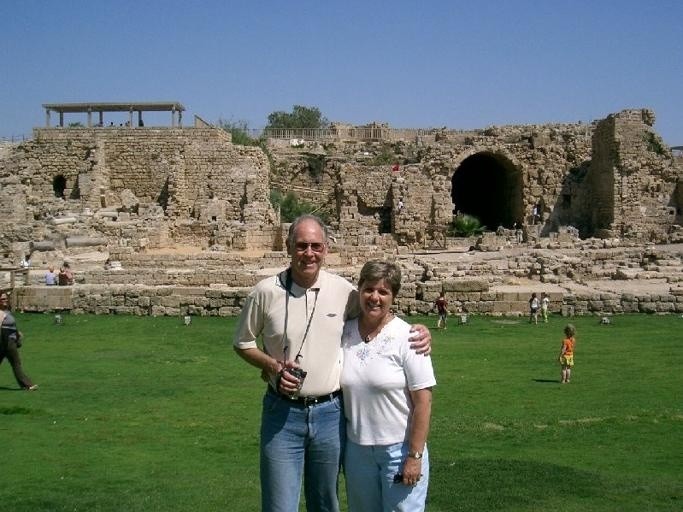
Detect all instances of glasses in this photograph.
[293,240,326,252]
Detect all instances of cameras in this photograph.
[287,368,307,401]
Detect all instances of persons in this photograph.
[44,260,74,286]
[20,255,33,272]
[528,292,540,325]
[227,214,433,512]
[539,291,549,324]
[558,323,576,384]
[455,305,471,325]
[124,121,130,127]
[95,122,105,127]
[435,291,452,329]
[395,198,403,213]
[107,122,114,127]
[116,123,124,128]
[335,258,438,512]
[0,292,39,391]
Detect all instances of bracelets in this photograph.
[407,450,423,459]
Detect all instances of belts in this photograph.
[267,383,342,405]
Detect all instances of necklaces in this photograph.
[353,315,389,345]
